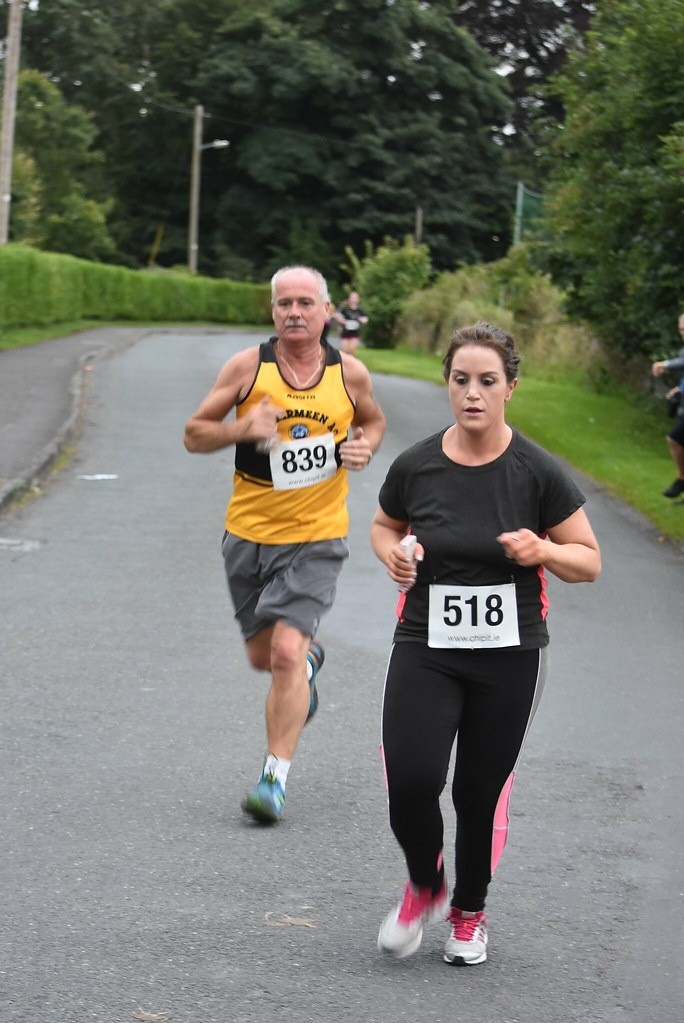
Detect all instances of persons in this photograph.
[653,314,684,497]
[335,291,368,353]
[183,264,385,821]
[373,322,602,964]
[321,292,336,341]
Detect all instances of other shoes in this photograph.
[662,478,684,498]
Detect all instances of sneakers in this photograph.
[443,906,488,967]
[378,880,450,959]
[241,751,285,822]
[303,641,325,728]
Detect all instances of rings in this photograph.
[512,536,521,542]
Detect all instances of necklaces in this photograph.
[278,339,322,387]
